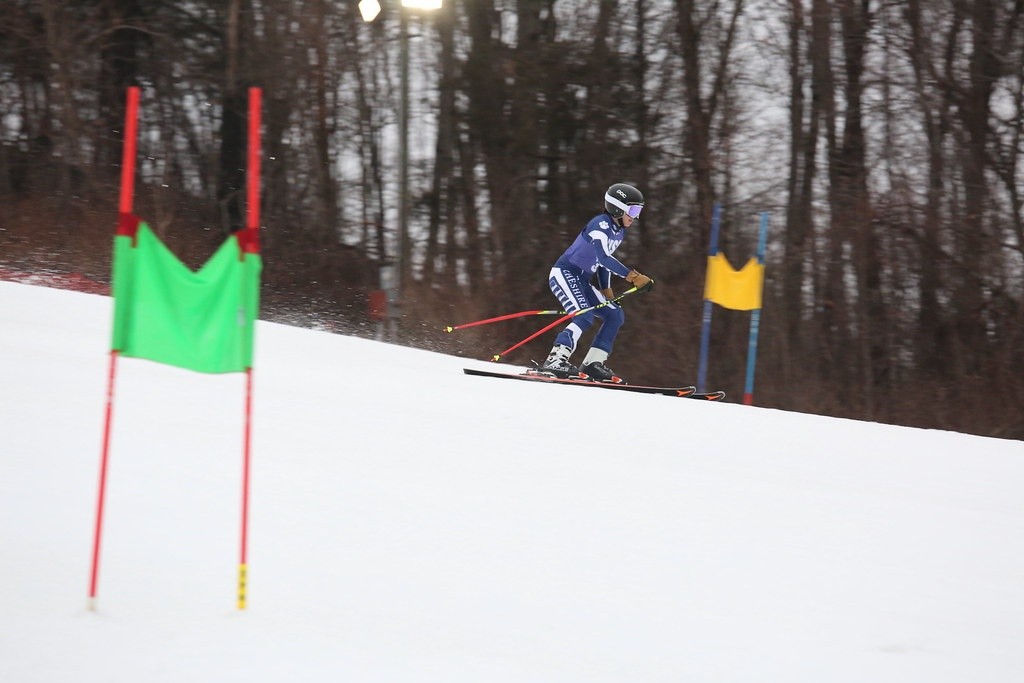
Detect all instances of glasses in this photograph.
[628,205,643,219]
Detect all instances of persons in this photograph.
[539,182,651,386]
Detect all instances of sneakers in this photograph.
[579,363,616,382]
[542,353,580,378]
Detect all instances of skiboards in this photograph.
[462,367,727,402]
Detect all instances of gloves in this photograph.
[625,269,655,296]
[601,289,623,308]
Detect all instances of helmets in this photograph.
[604,183,645,219]
